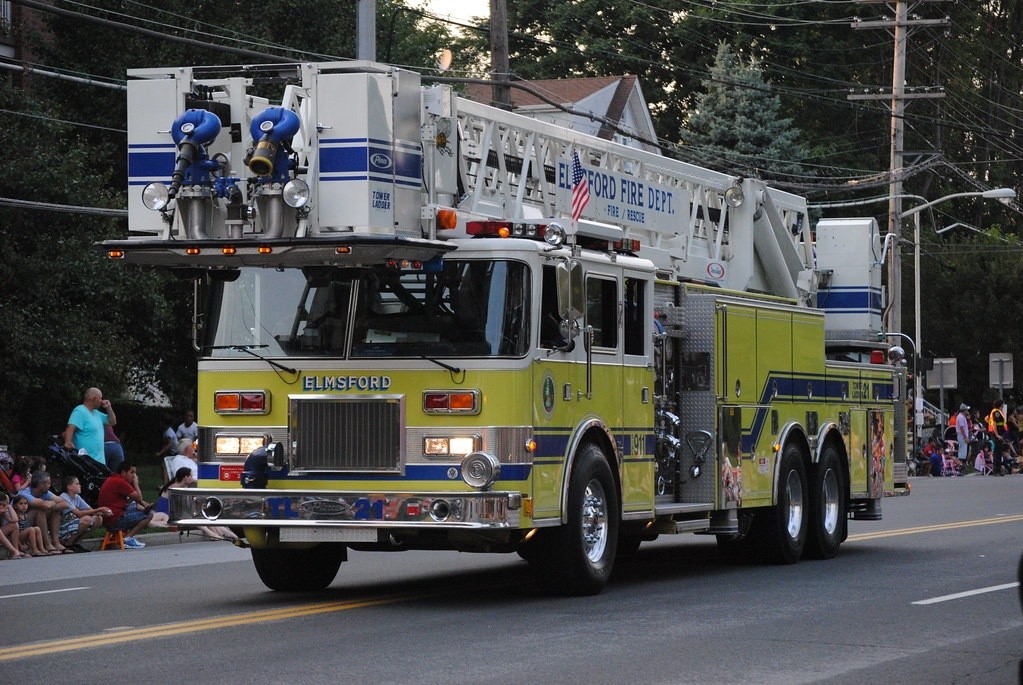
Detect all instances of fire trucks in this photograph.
[94,56,935,599]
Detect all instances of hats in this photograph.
[960,403,970,410]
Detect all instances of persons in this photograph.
[906,398,1023,477]
[0,386,238,560]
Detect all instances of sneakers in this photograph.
[124,537,145,549]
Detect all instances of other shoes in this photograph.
[20,553,32,558]
[75,544,90,552]
[32,552,45,557]
[42,551,52,555]
[994,473,1003,477]
[65,545,82,553]
[12,555,21,559]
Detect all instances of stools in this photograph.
[100,528,128,552]
[984,464,995,476]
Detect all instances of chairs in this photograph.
[941,454,958,477]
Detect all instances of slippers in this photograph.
[49,549,62,554]
[60,548,74,554]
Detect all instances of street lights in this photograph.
[888,188,1016,368]
